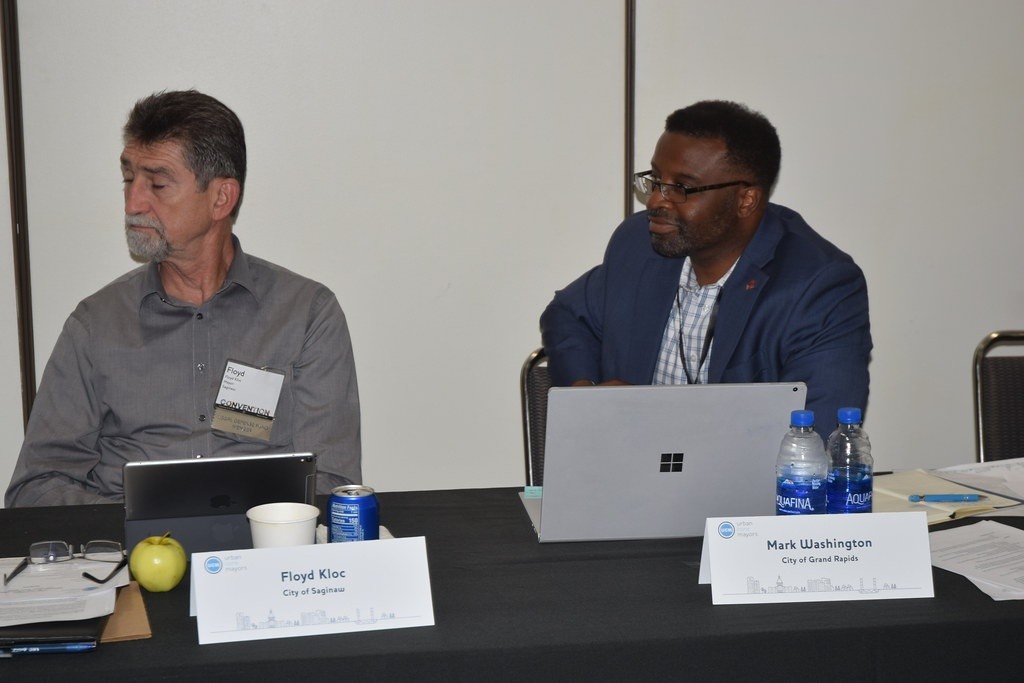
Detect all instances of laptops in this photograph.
[518,380,809,545]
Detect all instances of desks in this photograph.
[0,471,1024,683]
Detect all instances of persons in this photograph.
[540,100,874,451]
[4,88,362,508]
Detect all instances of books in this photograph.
[872,468,1024,525]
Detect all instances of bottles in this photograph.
[774,410,828,516]
[826,407,873,514]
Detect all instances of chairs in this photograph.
[972,330,1024,462]
[520,347,552,488]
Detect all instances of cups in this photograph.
[246,502,320,549]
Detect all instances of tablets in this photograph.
[123,452,318,522]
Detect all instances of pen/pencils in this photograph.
[908,493,988,502]
[11,644,96,654]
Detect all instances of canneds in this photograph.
[327,484,379,543]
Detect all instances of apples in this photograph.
[130,531,186,592]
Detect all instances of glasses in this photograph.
[2,539,130,586]
[632,169,754,203]
